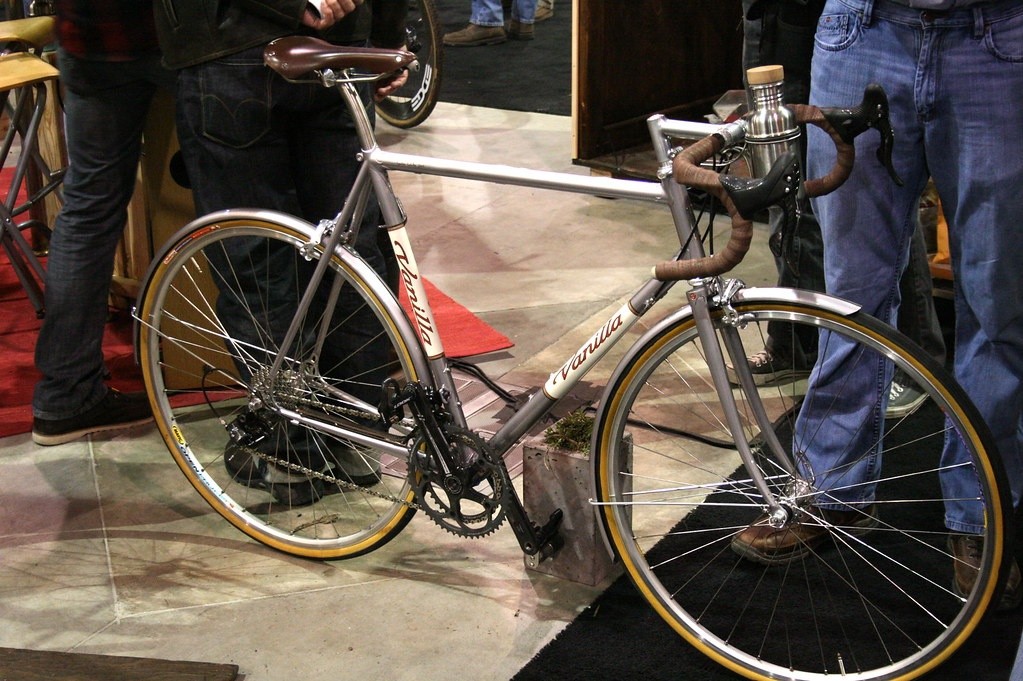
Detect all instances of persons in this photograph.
[29,0,422,516]
[715,0,951,419]
[440,0,537,48]
[726,0,1023,616]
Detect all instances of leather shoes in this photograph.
[731,505,880,566]
[946,530,1019,615]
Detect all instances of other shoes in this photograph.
[32,388,153,444]
[443,23,507,47]
[225,441,323,505]
[502,19,534,39]
[532,7,553,21]
[320,440,383,484]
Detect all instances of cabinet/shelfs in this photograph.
[33,47,246,390]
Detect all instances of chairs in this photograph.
[2,17,58,256]
[0,50,62,318]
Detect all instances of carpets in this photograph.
[0,166,513,441]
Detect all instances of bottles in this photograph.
[745,63,805,201]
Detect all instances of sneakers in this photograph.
[886,380,930,420]
[724,353,813,385]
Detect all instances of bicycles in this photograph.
[128,30,1013,681]
[352,2,442,129]
